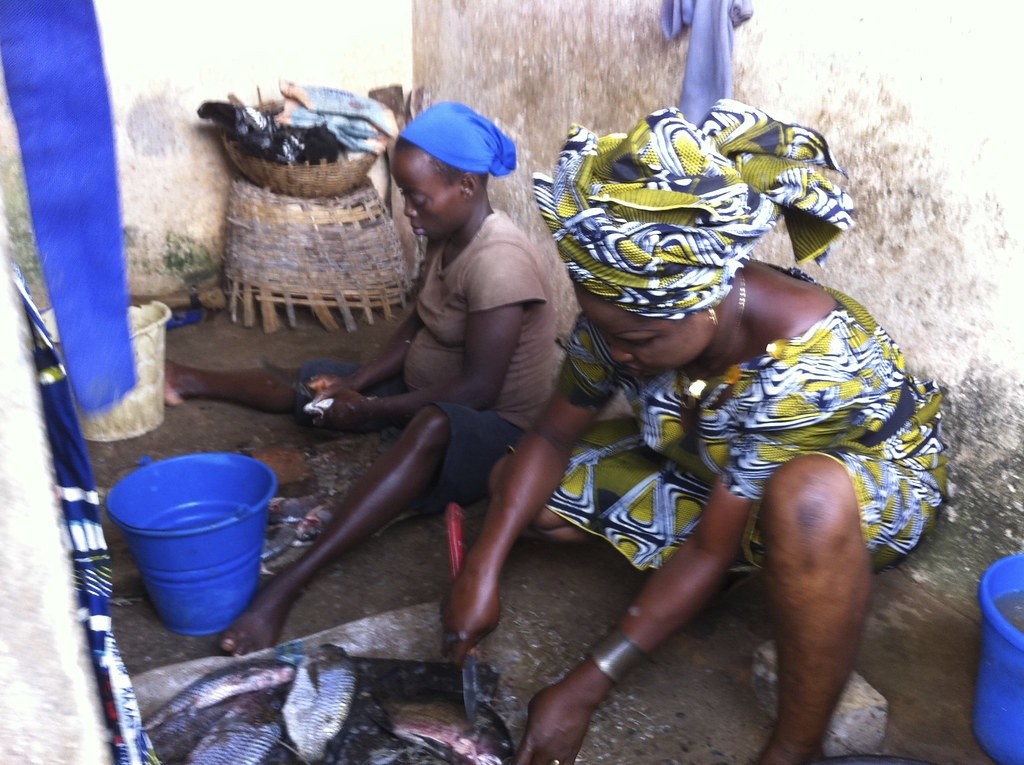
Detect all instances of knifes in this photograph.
[446,501,483,722]
[300,381,317,399]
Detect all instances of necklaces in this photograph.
[684,267,748,397]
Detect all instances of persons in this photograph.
[437,97,943,765]
[162,101,556,658]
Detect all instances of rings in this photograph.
[440,632,469,643]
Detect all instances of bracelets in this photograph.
[589,622,652,686]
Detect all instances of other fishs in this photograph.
[258,492,346,577]
[304,391,379,420]
[142,643,516,765]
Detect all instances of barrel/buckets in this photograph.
[35,297,172,445]
[966,550,1023,765]
[103,448,282,641]
